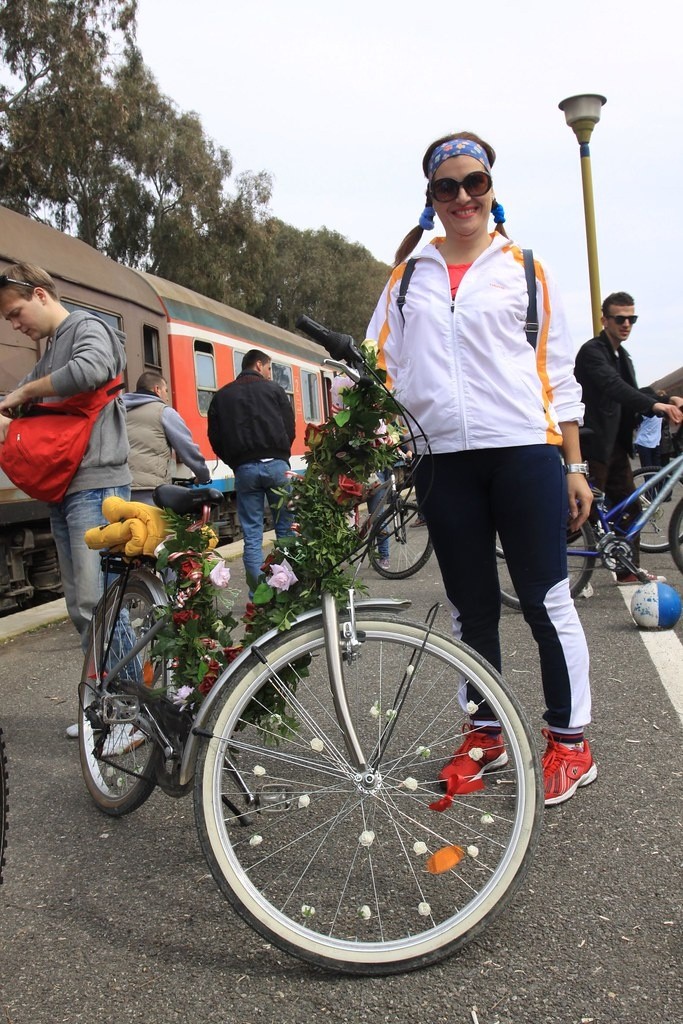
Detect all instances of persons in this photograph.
[575,291,682,587]
[207,349,300,609]
[367,131,600,810]
[366,411,426,572]
[0,263,211,757]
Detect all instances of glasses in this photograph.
[428,171,492,203]
[607,313,638,324]
[1,273,34,289]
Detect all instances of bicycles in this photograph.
[365,434,434,577]
[77,317,540,977]
[498,405,683,611]
[495,465,683,558]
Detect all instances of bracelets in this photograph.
[562,460,589,479]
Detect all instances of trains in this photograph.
[0,204,347,615]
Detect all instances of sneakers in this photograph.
[541,738,599,807]
[618,568,666,586]
[438,729,509,790]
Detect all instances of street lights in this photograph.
[557,94,607,338]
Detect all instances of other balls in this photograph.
[630,582,682,630]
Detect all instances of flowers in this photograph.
[146,344,415,751]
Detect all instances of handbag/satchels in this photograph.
[0,370,126,502]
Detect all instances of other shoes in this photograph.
[65,720,98,736]
[379,557,392,571]
[641,501,650,510]
[100,717,148,756]
